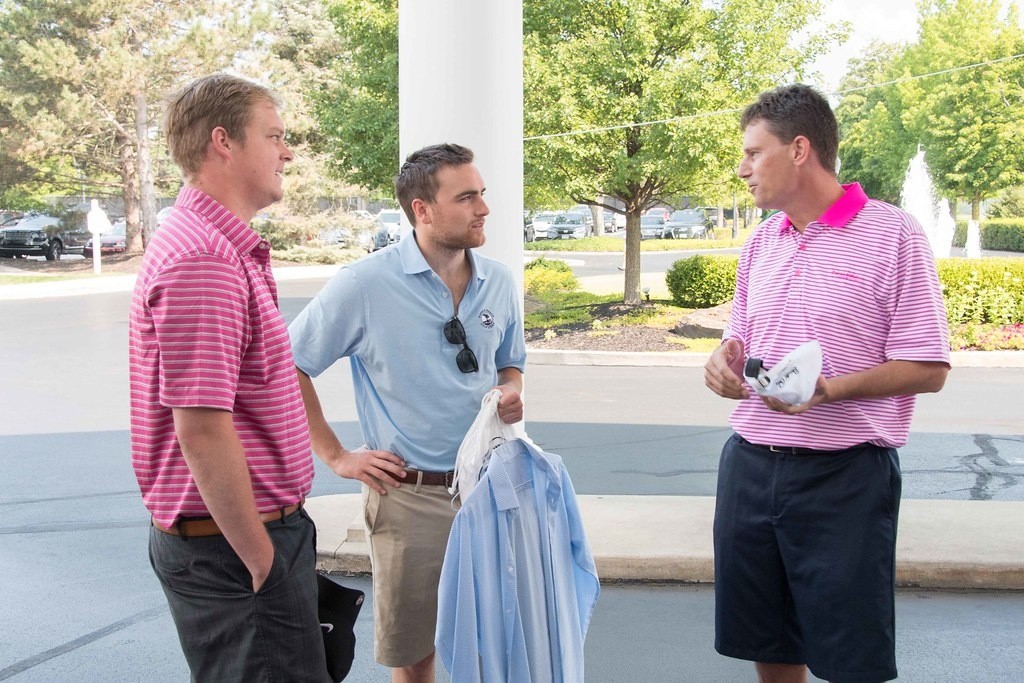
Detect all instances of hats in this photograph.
[317,572,365,682]
[743,339,822,407]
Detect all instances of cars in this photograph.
[0,217,24,226]
[579,209,594,231]
[644,208,671,222]
[141,206,174,249]
[0,217,64,261]
[532,215,559,242]
[615,209,626,229]
[338,219,390,253]
[83,220,145,257]
[375,208,401,243]
[320,210,374,246]
[61,232,92,255]
[548,213,589,240]
[603,211,615,233]
[664,209,712,239]
[640,216,665,240]
[703,207,727,227]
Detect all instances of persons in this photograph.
[129,72,333,683]
[705,84,951,683]
[287,143,524,683]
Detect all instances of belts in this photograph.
[741,440,821,455]
[386,468,454,488]
[151,494,305,536]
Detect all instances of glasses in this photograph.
[444,314,479,373]
